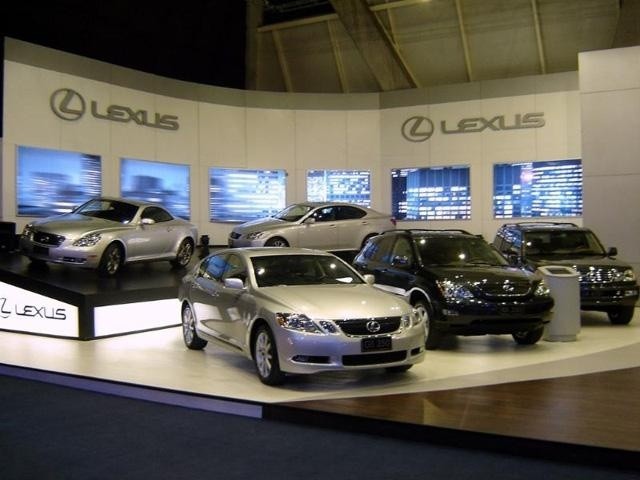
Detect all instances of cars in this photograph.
[18,198,198,278]
